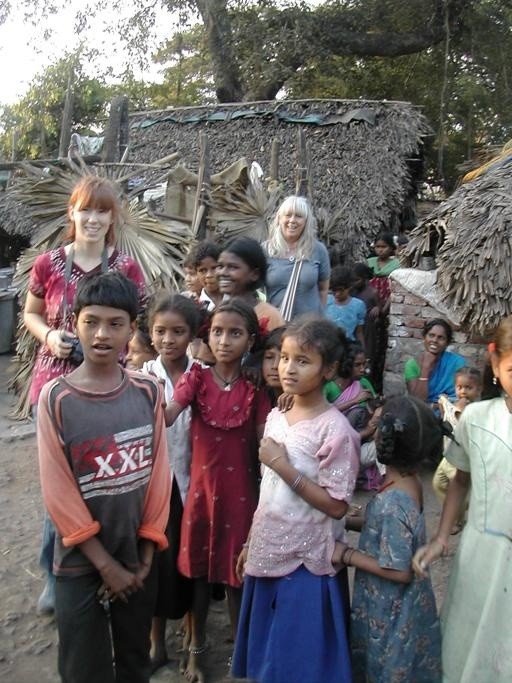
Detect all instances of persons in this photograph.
[36,273,171,682]
[22,177,148,611]
[404,316,512,683]
[264,196,330,323]
[128,237,452,683]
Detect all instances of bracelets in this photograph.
[44,327,55,346]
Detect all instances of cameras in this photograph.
[63,336,83,365]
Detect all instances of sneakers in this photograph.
[209,597,227,613]
[35,582,57,617]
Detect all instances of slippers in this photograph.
[450,519,467,535]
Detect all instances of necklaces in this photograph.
[285,246,300,261]
